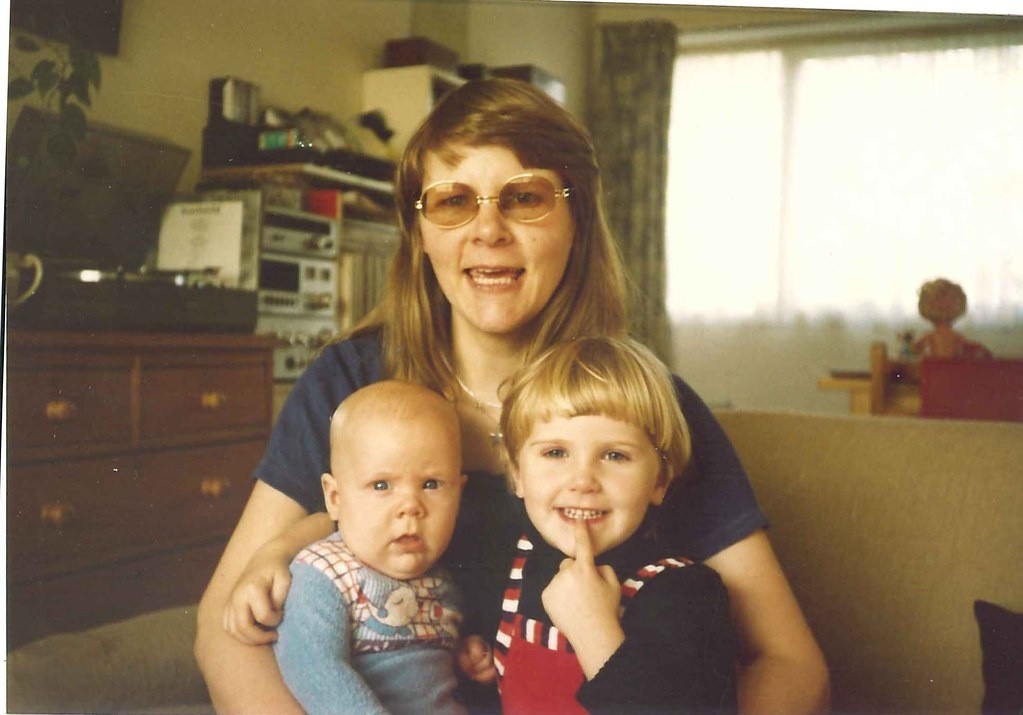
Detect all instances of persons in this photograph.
[194,80,832,715]
[226,337,741,715]
[274,379,497,715]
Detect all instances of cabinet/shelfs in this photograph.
[8,333,282,655]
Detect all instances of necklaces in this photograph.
[455,376,505,453]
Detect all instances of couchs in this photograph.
[6,409,1022,713]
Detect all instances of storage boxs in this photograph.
[384,37,566,104]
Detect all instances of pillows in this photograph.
[971,600,1022,713]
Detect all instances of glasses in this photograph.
[415,174,568,230]
